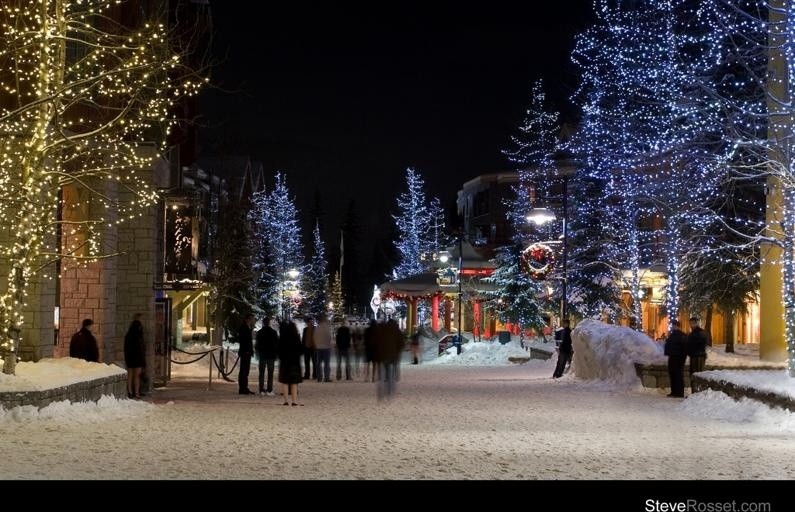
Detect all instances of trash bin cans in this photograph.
[499,332,510,345]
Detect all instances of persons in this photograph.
[133,312,147,397]
[552,319,572,378]
[689,318,713,394]
[124,320,144,400]
[664,319,688,398]
[227,311,419,407]
[71,318,100,364]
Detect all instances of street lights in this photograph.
[525,180,569,337]
[438,230,464,358]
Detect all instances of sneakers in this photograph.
[300,374,401,385]
[238,387,305,406]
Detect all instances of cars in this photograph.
[438,330,469,353]
